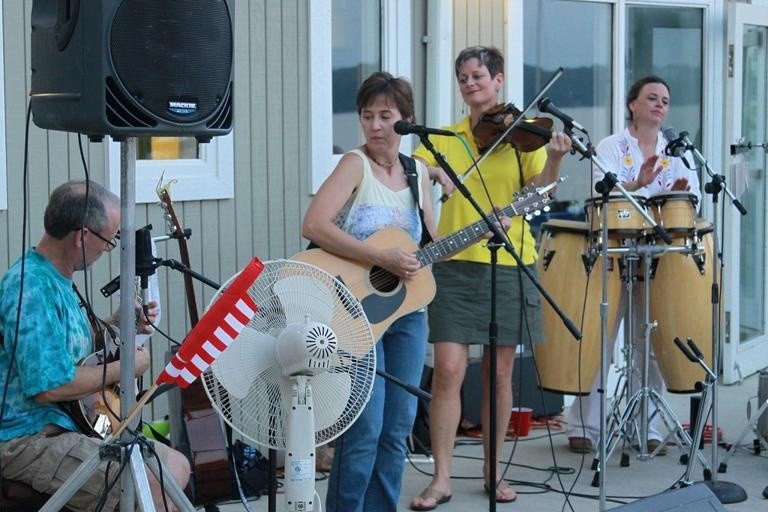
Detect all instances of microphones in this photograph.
[663,127,691,170]
[136,229,152,314]
[538,97,587,133]
[100,275,120,297]
[394,120,454,136]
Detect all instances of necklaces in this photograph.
[364,144,401,166]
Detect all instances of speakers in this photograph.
[601,482,728,511]
[461,350,563,428]
[30,0,235,143]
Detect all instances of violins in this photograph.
[473,102,577,155]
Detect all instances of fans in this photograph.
[196,256,379,512]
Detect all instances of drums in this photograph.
[649,192,698,232]
[533,219,624,396]
[584,195,648,238]
[626,218,724,395]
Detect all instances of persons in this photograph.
[410,45,574,510]
[300,70,513,512]
[0,178,193,511]
[566,77,703,456]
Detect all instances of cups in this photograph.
[511,406,533,437]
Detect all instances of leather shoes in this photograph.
[569,438,591,453]
[648,439,666,455]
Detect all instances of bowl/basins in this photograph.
[138,418,170,441]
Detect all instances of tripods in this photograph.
[36,138,198,512]
[592,254,712,487]
[591,258,687,471]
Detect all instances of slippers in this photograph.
[411,488,452,511]
[484,483,516,502]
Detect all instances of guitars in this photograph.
[158,191,234,505]
[278,179,564,365]
[75,293,144,439]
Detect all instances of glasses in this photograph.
[73,226,117,252]
